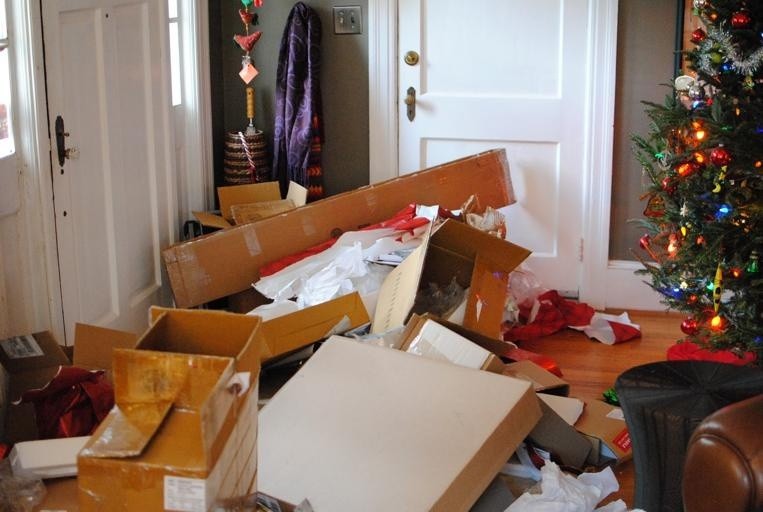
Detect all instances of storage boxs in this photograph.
[0,147,571,512]
